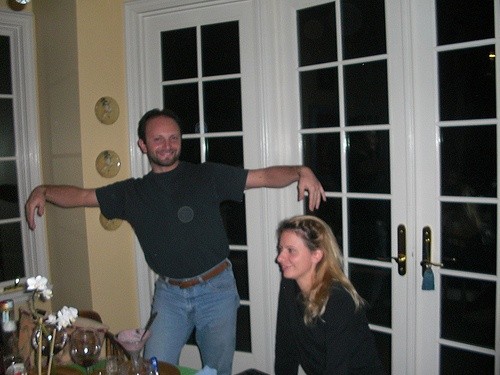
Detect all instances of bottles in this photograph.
[0,299,15,351]
[148,356,159,375]
[105,356,120,375]
[2,320,27,375]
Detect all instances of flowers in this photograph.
[26,275,78,341]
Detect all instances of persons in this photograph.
[25,109,326,375]
[275,215,385,375]
[439,158,493,271]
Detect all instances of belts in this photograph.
[159,263,228,289]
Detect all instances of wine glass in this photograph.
[114,328,152,375]
[32,322,68,375]
[69,327,102,375]
